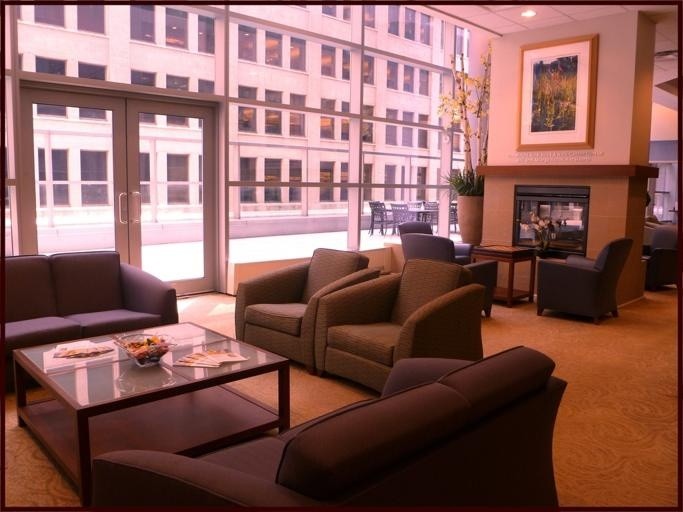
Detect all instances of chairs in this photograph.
[537,239,633,325]
[369,200,457,235]
[401,232,498,316]
[645,225,679,291]
[315,256,487,392]
[399,222,473,264]
[235,247,380,373]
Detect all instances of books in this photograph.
[171,348,247,368]
[53,340,114,359]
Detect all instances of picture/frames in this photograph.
[516,33,599,152]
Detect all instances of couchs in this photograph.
[92,343,568,506]
[5,251,178,392]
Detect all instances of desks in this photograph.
[470,244,537,307]
[12,321,290,506]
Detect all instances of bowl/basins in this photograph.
[113,333,178,368]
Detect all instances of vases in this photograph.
[457,195,483,245]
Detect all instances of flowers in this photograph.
[436,39,492,196]
[520,210,568,250]
